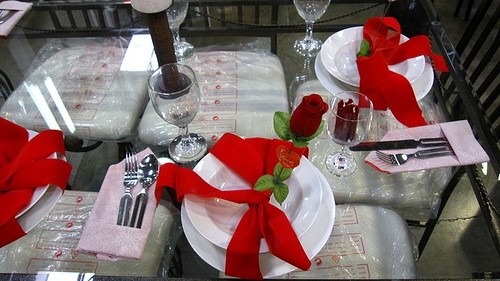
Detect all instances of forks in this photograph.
[115,150,139,226]
[376,147,455,165]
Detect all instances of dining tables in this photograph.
[0,0,500,281]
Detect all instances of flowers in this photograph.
[252,94,329,206]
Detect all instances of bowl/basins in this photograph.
[184,153,323,253]
[321,26,425,88]
[13,130,58,221]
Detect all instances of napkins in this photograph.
[364,120,490,173]
[76,146,163,260]
[0,0,34,36]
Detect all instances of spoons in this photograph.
[130,153,158,230]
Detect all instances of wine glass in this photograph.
[293,0,331,56]
[325,91,373,177]
[148,63,209,165]
[152,0,195,61]
[289,57,316,107]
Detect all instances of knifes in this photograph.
[349,136,449,150]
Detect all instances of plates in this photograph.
[314,51,435,107]
[19,152,69,234]
[180,153,337,278]
